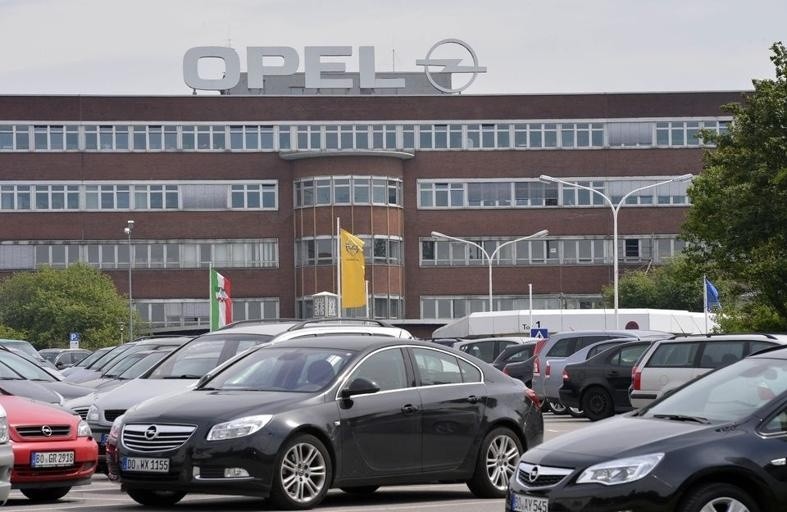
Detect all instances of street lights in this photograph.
[430,229,549,312]
[123,219,134,342]
[538,173,693,309]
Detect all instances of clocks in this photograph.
[313,290,342,319]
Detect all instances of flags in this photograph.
[705,276,721,310]
[337,228,368,308]
[209,265,232,331]
